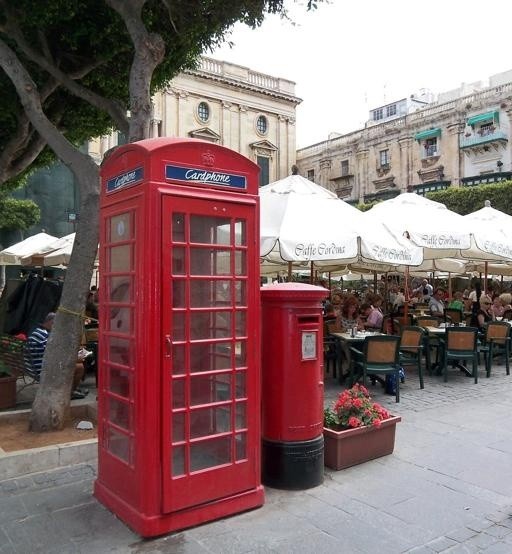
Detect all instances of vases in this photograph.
[322,414,400,470]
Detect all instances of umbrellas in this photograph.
[0,230,101,276]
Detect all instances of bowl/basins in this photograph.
[496,316,503,321]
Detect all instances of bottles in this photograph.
[491,311,495,322]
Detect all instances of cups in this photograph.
[440,320,466,326]
[503,318,508,322]
[353,327,357,336]
[349,327,355,337]
[360,330,365,335]
[421,309,424,315]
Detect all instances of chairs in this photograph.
[0,335,40,396]
[324,300,512,405]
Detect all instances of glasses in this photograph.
[482,302,491,305]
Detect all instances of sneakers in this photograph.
[71,387,89,400]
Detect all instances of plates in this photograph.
[356,335,370,337]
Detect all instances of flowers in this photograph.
[322,382,393,430]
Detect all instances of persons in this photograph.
[25,285,101,399]
